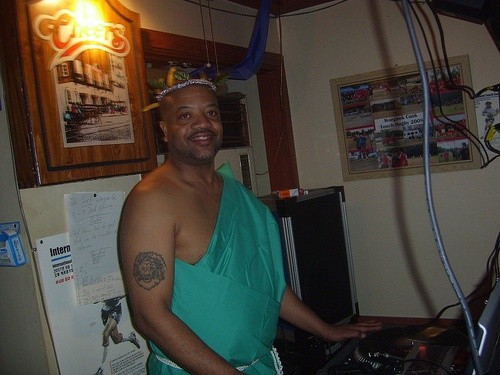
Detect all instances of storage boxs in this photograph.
[252,186,361,352]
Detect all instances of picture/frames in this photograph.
[330,54,483,182]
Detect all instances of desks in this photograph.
[284,315,500,375]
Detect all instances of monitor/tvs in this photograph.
[257,185,359,365]
[464,278,500,375]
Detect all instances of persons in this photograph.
[65,102,129,126]
[117,74,382,375]
[94,297,140,375]
[480,101,498,139]
[345,75,469,170]
